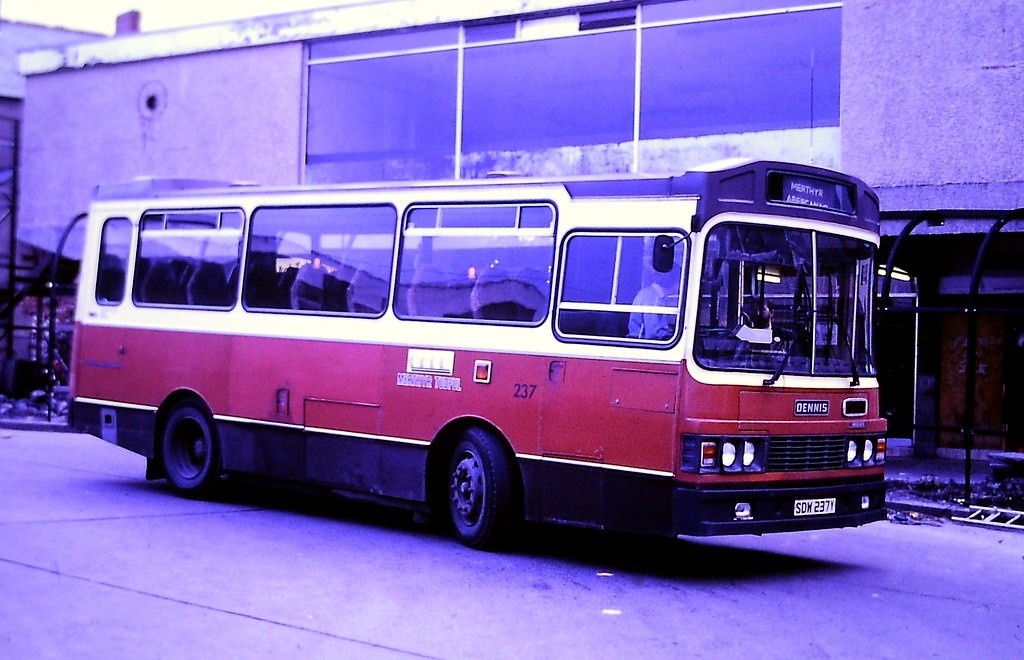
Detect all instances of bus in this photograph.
[62,154,888,556]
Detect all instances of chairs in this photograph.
[113,244,652,346]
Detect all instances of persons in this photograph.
[437,279,475,320]
[746,297,795,344]
[625,262,685,342]
[510,267,553,324]
